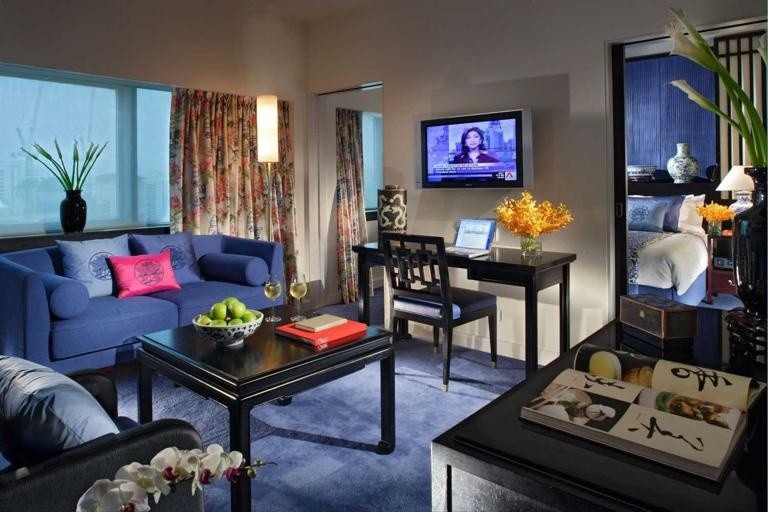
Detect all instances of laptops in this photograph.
[432,216,496,259]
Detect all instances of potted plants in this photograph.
[494,191,575,261]
[19,138,109,234]
[696,200,736,237]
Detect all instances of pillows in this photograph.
[54,233,132,299]
[130,231,205,285]
[628,197,672,232]
[38,271,90,320]
[0,354,120,465]
[198,252,270,287]
[651,194,687,233]
[107,248,182,298]
[678,193,708,248]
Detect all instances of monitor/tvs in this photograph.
[413,107,536,191]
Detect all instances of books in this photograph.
[274,312,369,347]
[519,342,768,483]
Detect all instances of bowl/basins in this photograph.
[192,308,264,347]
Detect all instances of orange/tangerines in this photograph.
[210,303,226,317]
[213,321,226,329]
[197,316,208,324]
[231,322,244,327]
[244,314,257,324]
[223,298,240,308]
[230,304,246,317]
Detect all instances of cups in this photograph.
[290,273,308,322]
[264,276,281,323]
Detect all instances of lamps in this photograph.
[715,165,755,192]
[255,94,279,242]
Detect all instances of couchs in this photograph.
[0,234,285,375]
[0,368,205,512]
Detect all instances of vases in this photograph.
[725,167,768,370]
[628,164,657,183]
[667,142,700,184]
[729,192,753,224]
[376,184,408,242]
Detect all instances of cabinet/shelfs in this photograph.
[430,304,768,512]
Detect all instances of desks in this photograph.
[351,241,577,379]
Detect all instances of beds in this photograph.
[626,181,720,306]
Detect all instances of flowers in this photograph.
[665,5,768,168]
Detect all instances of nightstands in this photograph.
[706,229,738,304]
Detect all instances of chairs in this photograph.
[380,231,499,392]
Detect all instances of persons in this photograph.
[448,126,499,164]
